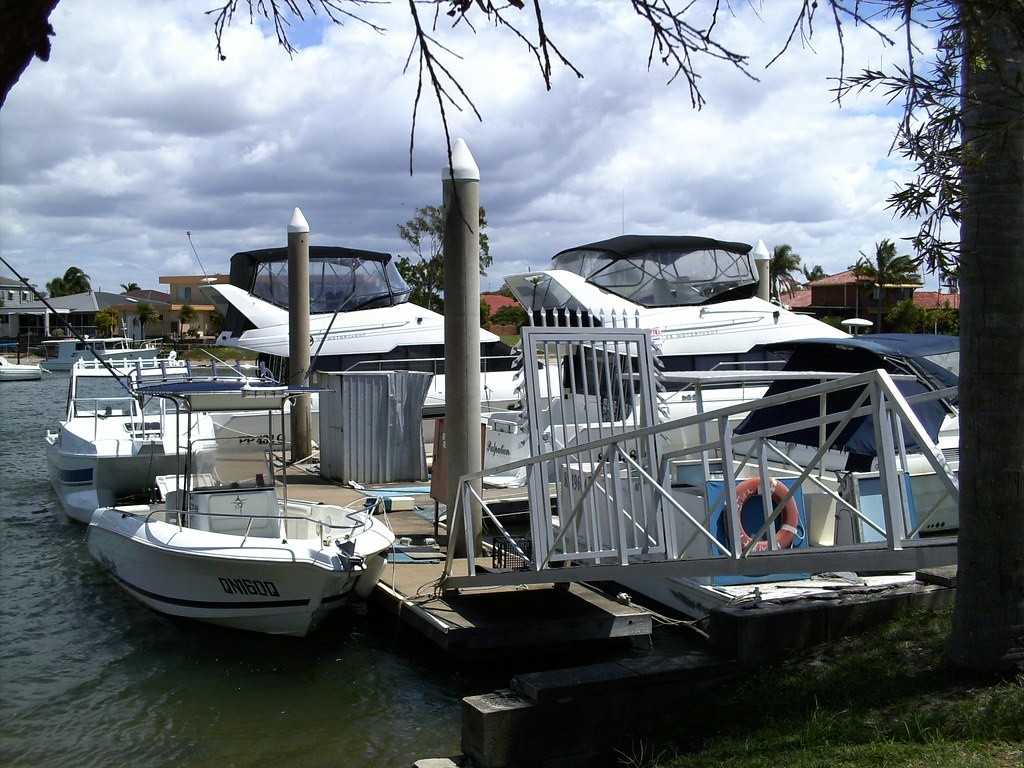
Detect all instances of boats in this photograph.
[45,356,219,526]
[206,231,962,539]
[0,356,42,381]
[41,317,164,371]
[83,364,395,639]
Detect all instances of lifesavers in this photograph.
[725,478,798,554]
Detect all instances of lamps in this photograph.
[239,377,254,392]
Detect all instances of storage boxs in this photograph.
[493,535,532,572]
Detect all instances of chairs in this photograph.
[652,276,707,304]
